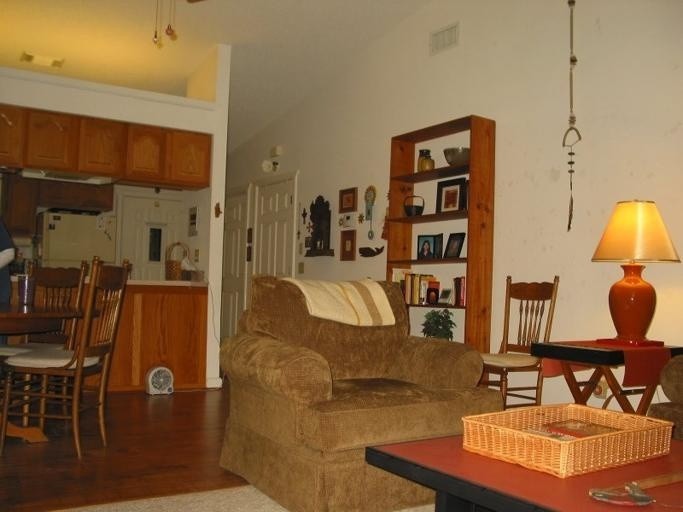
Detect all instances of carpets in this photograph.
[44,482,441,511]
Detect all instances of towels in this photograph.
[283,276,396,329]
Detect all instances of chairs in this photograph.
[215,276,506,512]
[475,276,563,410]
[0,255,130,462]
[644,355,682,440]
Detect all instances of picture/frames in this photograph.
[338,186,358,213]
[416,234,436,260]
[442,232,466,259]
[338,228,356,262]
[434,176,466,213]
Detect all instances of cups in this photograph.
[18,275,36,304]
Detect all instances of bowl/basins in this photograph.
[181,270,204,281]
[443,148,469,167]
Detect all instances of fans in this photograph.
[144,367,175,397]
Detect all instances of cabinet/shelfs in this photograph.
[112,121,210,195]
[3,277,207,395]
[0,103,28,170]
[0,173,38,239]
[383,111,494,358]
[25,104,129,184]
[38,178,113,215]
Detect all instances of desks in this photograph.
[530,335,682,417]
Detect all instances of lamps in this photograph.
[592,197,680,348]
[150,0,161,43]
[165,0,175,36]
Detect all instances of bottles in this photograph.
[417,149,430,172]
[421,155,434,171]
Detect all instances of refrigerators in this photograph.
[37,213,116,269]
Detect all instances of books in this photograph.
[391,268,466,306]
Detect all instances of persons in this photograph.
[448,240,458,257]
[0,223,16,378]
[419,240,433,260]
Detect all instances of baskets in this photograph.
[459,400,676,481]
[163,241,192,282]
